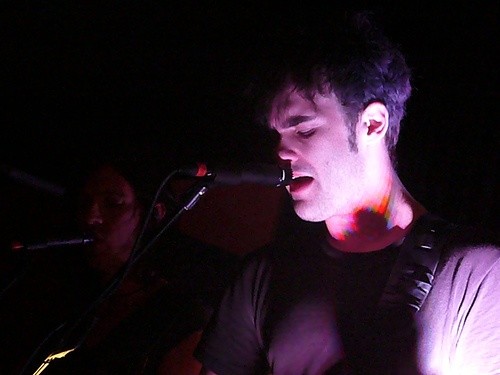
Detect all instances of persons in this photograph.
[190,14,499,375]
[7,135,244,375]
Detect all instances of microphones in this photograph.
[11,232,95,253]
[180,163,292,187]
